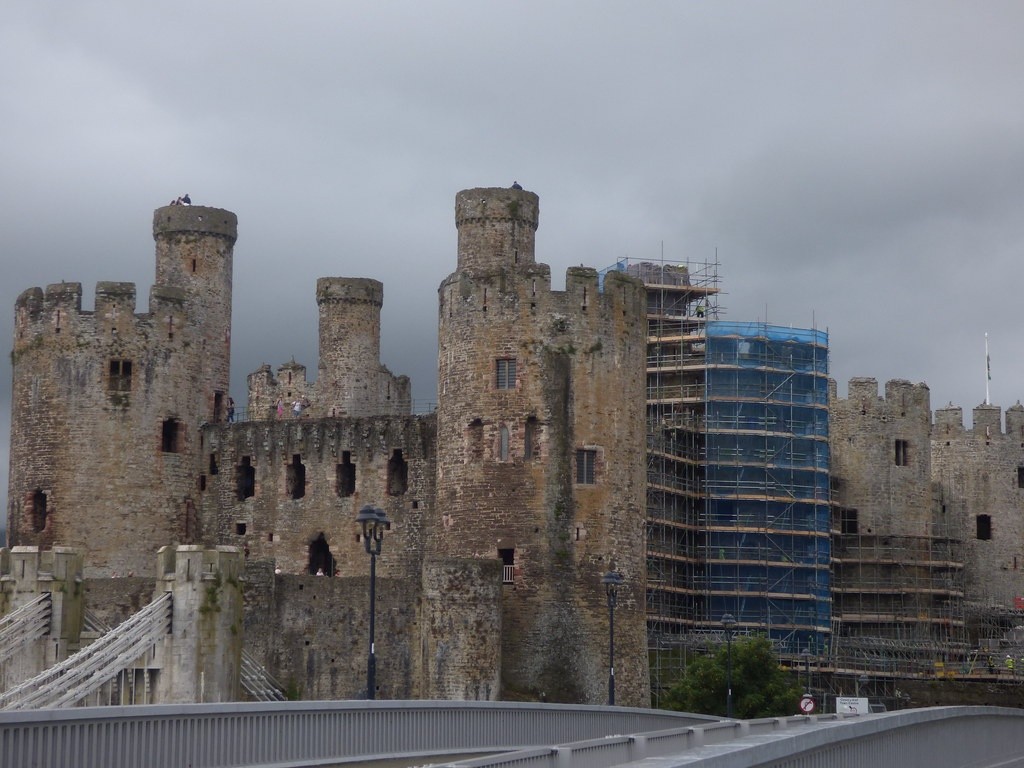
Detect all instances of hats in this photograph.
[1006,655,1010,658]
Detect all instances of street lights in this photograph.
[801,648,812,716]
[720,613,737,717]
[600,571,623,706]
[355,504,390,701]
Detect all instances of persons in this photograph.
[316,568,324,575]
[276,398,286,419]
[243,545,250,558]
[511,180,523,189]
[718,548,725,559]
[987,656,1001,674]
[169,200,175,206]
[175,197,182,205]
[225,397,235,422]
[291,397,302,418]
[807,634,816,649]
[181,194,191,205]
[1004,655,1015,674]
[696,305,705,318]
